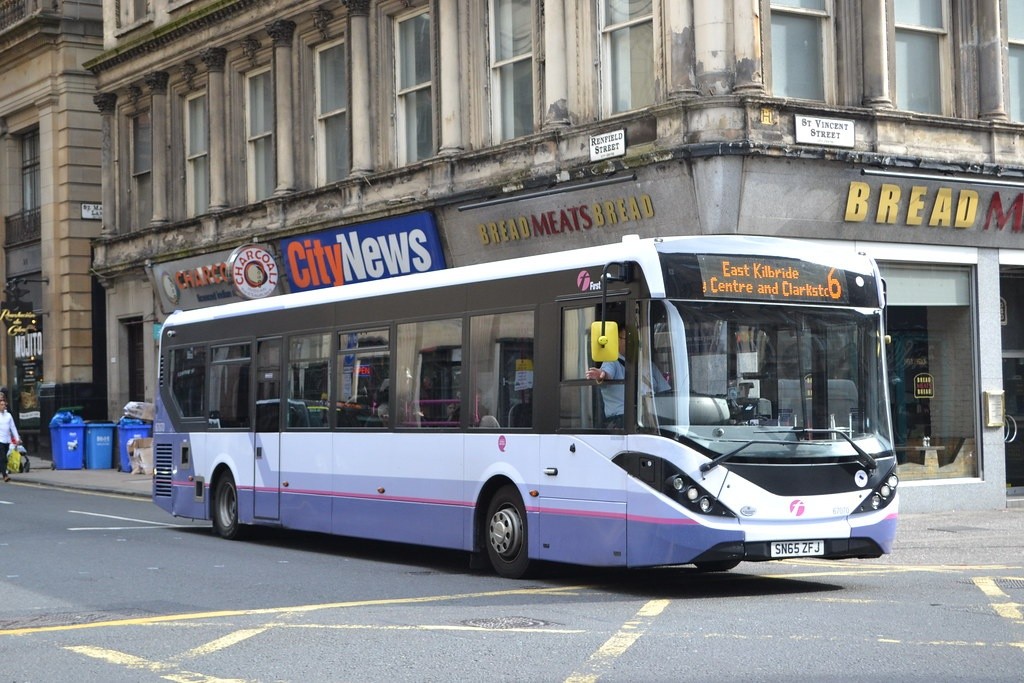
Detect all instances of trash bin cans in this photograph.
[117,424,152,472]
[49,423,86,470]
[85,423,117,469]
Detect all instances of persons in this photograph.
[586,325,672,429]
[0,393,23,483]
[378,374,486,428]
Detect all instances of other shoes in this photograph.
[3,474,10,482]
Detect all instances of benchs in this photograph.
[894,436,979,481]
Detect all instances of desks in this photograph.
[894,446,945,467]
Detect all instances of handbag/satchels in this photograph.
[6,446,22,472]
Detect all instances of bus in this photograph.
[653,316,862,438]
[153,235,900,581]
